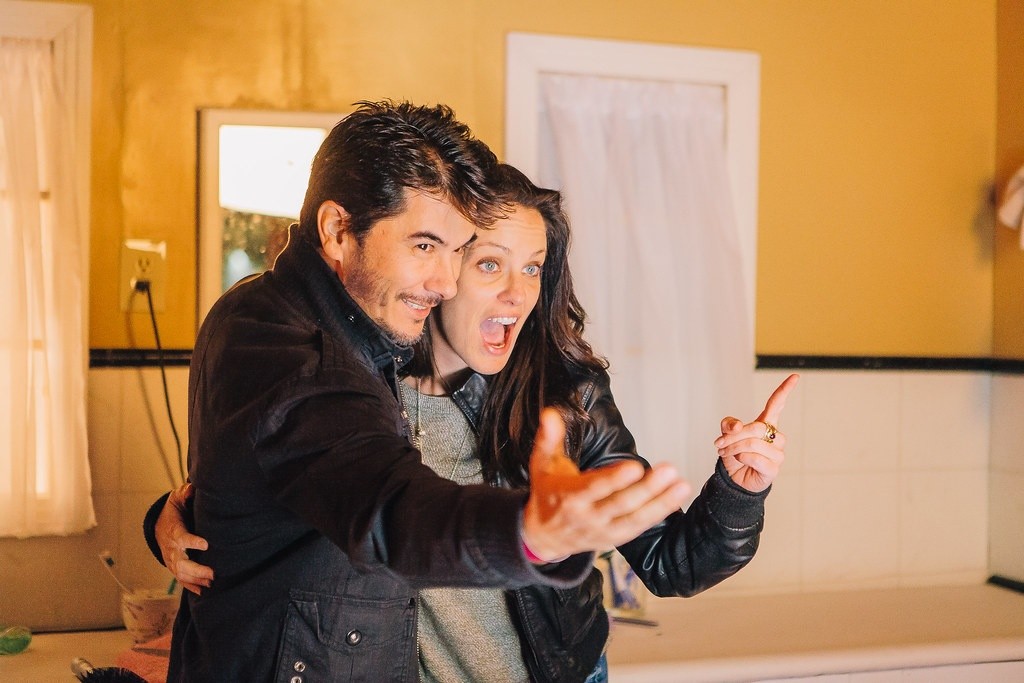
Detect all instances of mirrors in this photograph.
[194,108,357,344]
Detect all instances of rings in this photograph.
[760,421,778,446]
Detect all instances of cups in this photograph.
[122,585,178,645]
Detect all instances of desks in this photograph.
[2,580,1023,682]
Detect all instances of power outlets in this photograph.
[118,246,167,313]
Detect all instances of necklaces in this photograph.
[417,376,473,481]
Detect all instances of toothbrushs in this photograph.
[97,550,140,599]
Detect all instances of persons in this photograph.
[167,98,696,682]
[138,160,800,683]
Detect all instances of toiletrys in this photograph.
[1,624,33,658]
